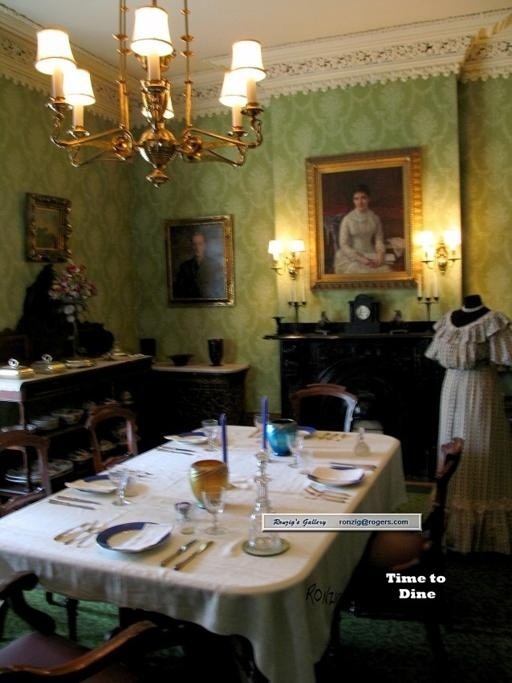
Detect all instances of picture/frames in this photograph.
[25,194,71,261]
[163,214,235,308]
[305,144,425,291]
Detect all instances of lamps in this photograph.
[34,0,270,186]
[267,240,306,280]
[416,226,462,273]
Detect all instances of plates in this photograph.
[1,406,123,486]
[61,431,364,552]
[3,452,77,484]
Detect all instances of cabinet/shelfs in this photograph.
[263,321,443,484]
[0,353,152,499]
[152,362,250,445]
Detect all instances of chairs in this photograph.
[0,431,52,517]
[290,386,358,431]
[85,406,137,471]
[333,437,466,668]
[0,572,158,683]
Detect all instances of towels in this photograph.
[163,436,208,443]
[296,461,365,481]
[64,479,118,494]
[112,522,174,552]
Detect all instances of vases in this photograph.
[53,302,87,358]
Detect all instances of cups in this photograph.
[207,338,225,366]
[253,414,297,456]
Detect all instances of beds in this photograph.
[0,424,409,683]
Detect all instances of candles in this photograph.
[287,279,306,303]
[417,272,440,300]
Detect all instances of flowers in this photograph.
[49,263,97,320]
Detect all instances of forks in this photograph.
[48,518,107,547]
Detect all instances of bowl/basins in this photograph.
[167,353,194,366]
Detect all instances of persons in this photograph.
[331,182,393,273]
[421,293,510,558]
[173,229,225,297]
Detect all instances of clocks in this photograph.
[346,294,382,333]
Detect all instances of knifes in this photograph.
[158,536,214,571]
[46,493,105,511]
[327,459,382,472]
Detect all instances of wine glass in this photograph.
[200,417,219,453]
